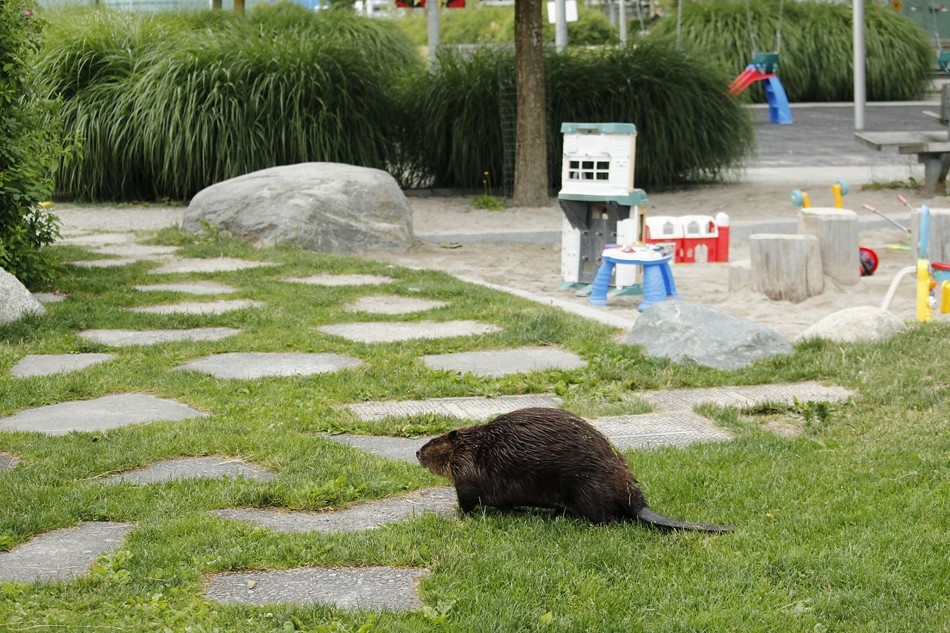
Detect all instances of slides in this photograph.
[729,66,761,99]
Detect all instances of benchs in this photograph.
[851,81,950,199]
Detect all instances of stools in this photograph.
[588,241,682,315]
